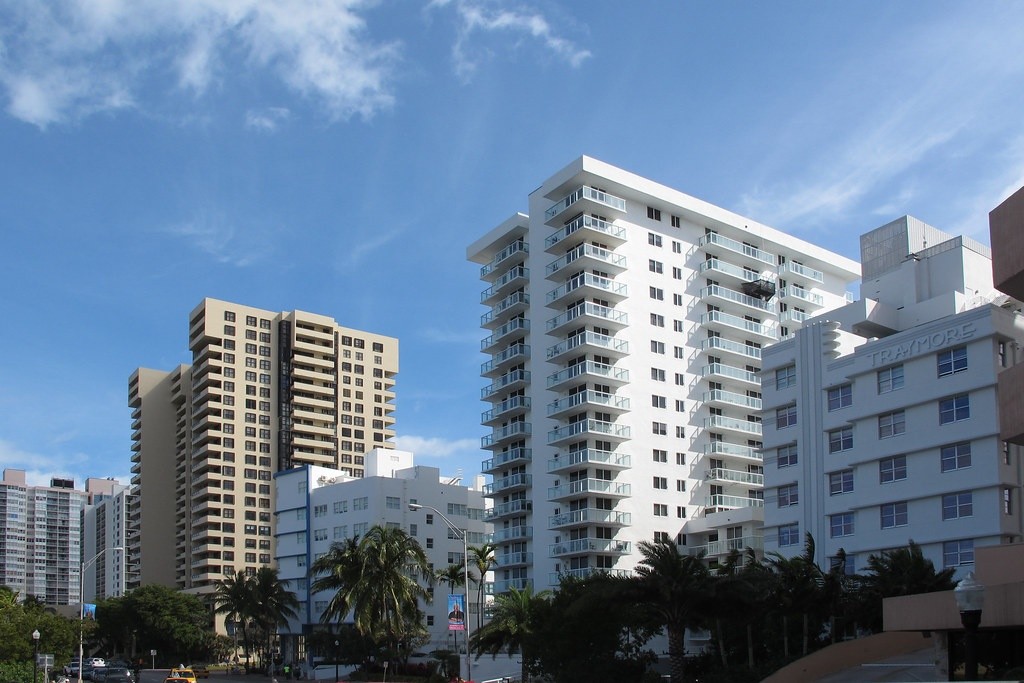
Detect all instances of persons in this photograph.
[448,604,464,624]
[84,610,94,620]
[283,657,305,681]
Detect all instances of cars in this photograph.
[64,657,137,683]
[169,664,198,683]
[186,663,210,679]
[162,673,192,683]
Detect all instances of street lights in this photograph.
[33,629,41,683]
[953,570,989,682]
[407,504,472,681]
[334,640,340,681]
[79,546,125,683]
